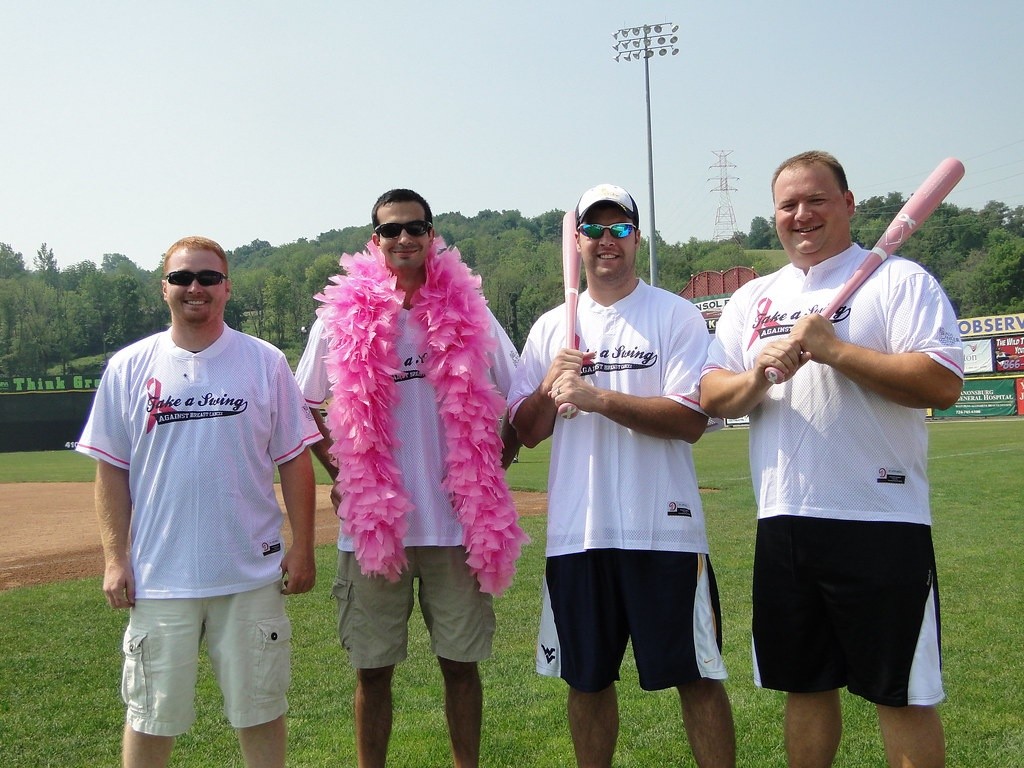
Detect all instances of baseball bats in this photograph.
[764,158,967,384]
[555,211,587,419]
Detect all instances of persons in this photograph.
[700,151,965,768]
[75,239,323,768]
[507,183,736,768]
[295,189,521,768]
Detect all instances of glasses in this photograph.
[375,220,433,238]
[164,269,231,287]
[576,223,637,238]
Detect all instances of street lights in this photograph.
[611,16,679,289]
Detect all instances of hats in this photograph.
[574,184,639,231]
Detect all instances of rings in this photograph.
[556,387,560,395]
